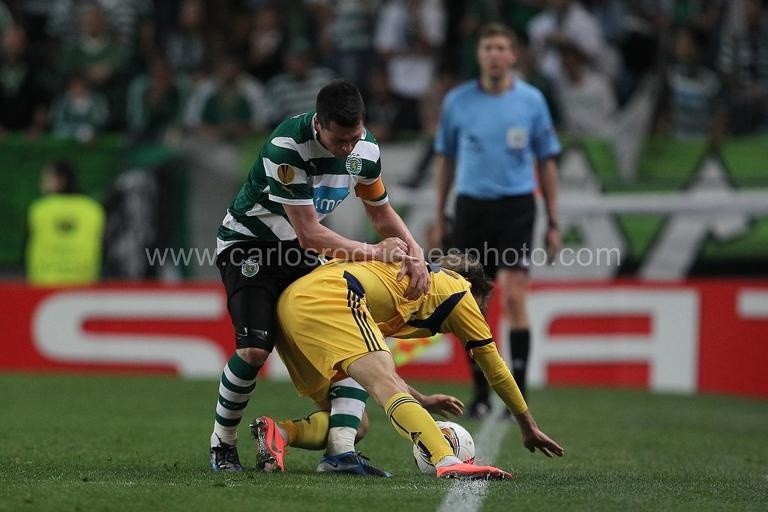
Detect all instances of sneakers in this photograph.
[467,399,492,420]
[316,449,394,478]
[435,462,514,481]
[249,415,289,474]
[208,431,249,474]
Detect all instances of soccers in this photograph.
[412,422,474,475]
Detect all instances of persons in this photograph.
[209,81,432,474]
[430,22,563,422]
[248,249,564,480]
